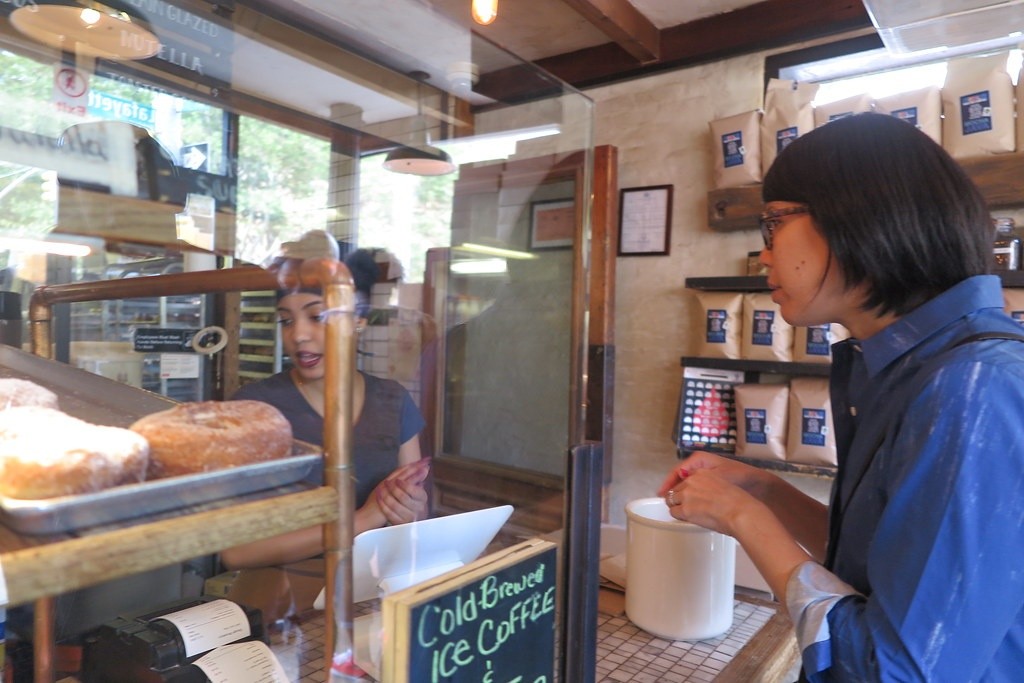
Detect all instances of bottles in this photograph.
[746,251,768,276]
[992,217,1021,272]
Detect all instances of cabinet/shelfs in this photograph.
[0,260,357,683]
[674,147,1022,606]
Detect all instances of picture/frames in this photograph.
[526,197,577,250]
[618,184,673,254]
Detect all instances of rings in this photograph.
[670,490,676,505]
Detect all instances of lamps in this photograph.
[382,70,453,173]
[1,0,160,63]
[472,1,497,25]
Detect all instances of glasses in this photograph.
[754,205,811,251]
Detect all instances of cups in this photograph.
[625,498,736,641]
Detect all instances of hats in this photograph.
[277,239,371,319]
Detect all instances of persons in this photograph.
[245,573,389,682]
[440,156,595,474]
[218,232,430,572]
[657,111,1024,683]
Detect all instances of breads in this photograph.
[0,379,292,499]
[131,311,274,356]
